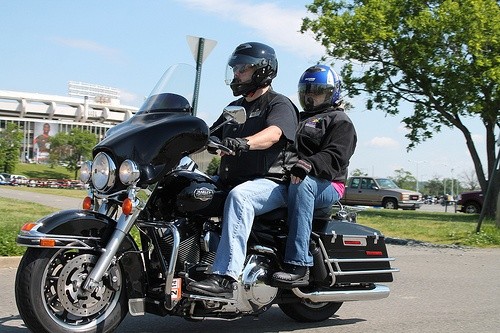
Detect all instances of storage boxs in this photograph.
[310,220,393,282]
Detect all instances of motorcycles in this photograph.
[11,61,402,332]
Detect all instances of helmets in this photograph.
[298,64,340,112]
[227,41,278,97]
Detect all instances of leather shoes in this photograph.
[272,267,311,285]
[186,275,233,299]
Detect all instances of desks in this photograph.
[443,200,458,214]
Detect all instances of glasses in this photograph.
[231,64,256,73]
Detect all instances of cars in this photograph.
[339,175,423,210]
[1,155,92,190]
[455,179,495,215]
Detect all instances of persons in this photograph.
[271,64,358,284]
[186,42,300,300]
[34,123,53,152]
